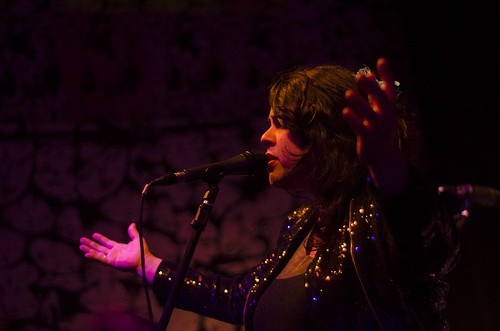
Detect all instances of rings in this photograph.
[102,254,107,259]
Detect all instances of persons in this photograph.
[80,57,455,331]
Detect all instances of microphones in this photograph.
[438,185,500,207]
[150,150,269,186]
